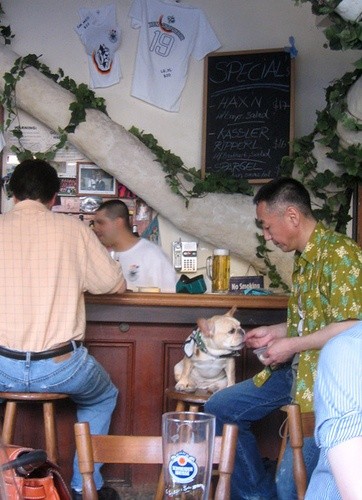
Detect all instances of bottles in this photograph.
[132,223,139,238]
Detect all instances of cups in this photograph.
[206,248,230,295]
[161,411,216,500]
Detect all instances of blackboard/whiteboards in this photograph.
[200,44,298,183]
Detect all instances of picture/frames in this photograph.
[76,162,119,198]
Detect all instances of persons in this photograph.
[0,159,126,500]
[203,177,362,500]
[303,322,362,500]
[92,200,181,294]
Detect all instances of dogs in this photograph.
[173,304,248,396]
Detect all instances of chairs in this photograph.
[278,405,316,499]
[73,421,238,500]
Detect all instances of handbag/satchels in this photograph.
[0,444,73,500]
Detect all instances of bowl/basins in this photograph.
[254,347,267,362]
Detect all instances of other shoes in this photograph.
[74,486,119,500]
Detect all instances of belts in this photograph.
[0,341,81,360]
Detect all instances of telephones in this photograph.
[172,237,197,273]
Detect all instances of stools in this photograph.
[154,385,222,500]
[0,392,71,467]
